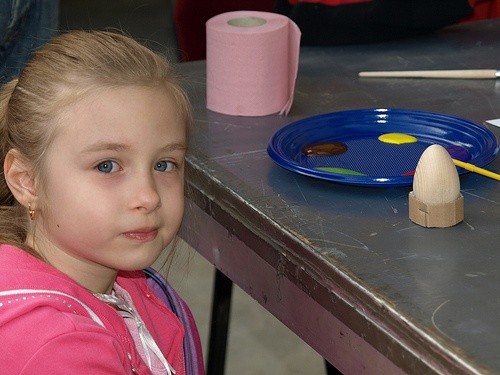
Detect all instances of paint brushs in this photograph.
[358,68,500,80]
[450,157,500,180]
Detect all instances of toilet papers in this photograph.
[205,10,301,116]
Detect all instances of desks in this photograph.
[126,32,500,375]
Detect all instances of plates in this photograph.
[266,107,500,189]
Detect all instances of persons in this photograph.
[1,29,208,375]
[275,1,500,48]
